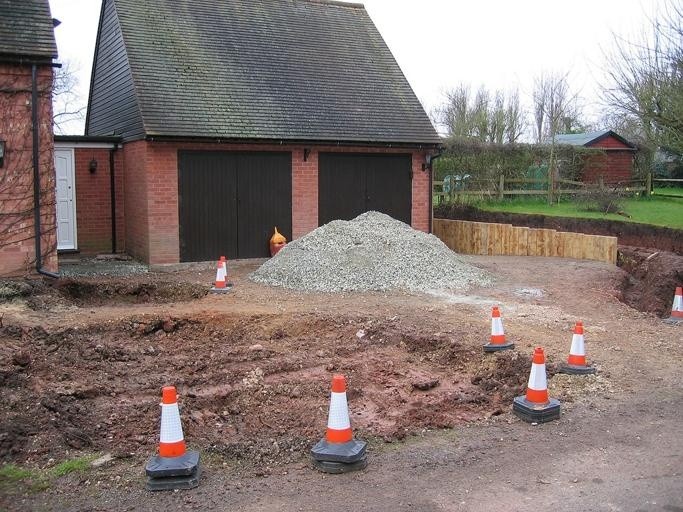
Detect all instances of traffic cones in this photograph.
[662,287,683,324]
[210,254,235,294]
[562,322,597,374]
[142,386,203,493]
[485,304,516,354]
[308,374,371,475]
[510,347,561,426]
